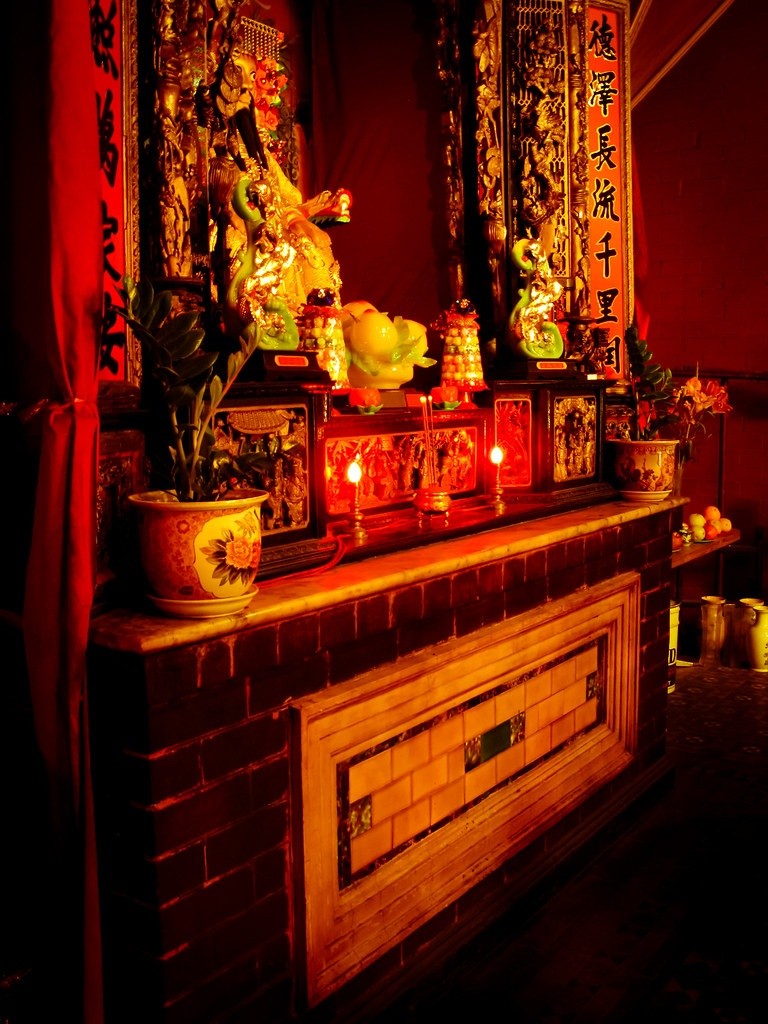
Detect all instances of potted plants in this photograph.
[606,323,680,491]
[108,275,286,601]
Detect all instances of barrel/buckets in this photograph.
[666,600,681,695]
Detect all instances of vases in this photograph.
[701,596,768,673]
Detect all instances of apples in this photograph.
[687,513,718,540]
[672,531,683,549]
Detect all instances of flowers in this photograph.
[681,362,733,464]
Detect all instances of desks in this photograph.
[671,528,741,603]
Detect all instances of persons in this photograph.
[212,15,351,351]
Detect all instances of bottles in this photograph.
[698,595,768,673]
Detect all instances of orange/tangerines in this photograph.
[702,506,732,534]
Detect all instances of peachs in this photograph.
[338,299,427,355]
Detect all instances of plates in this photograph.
[694,539,717,543]
